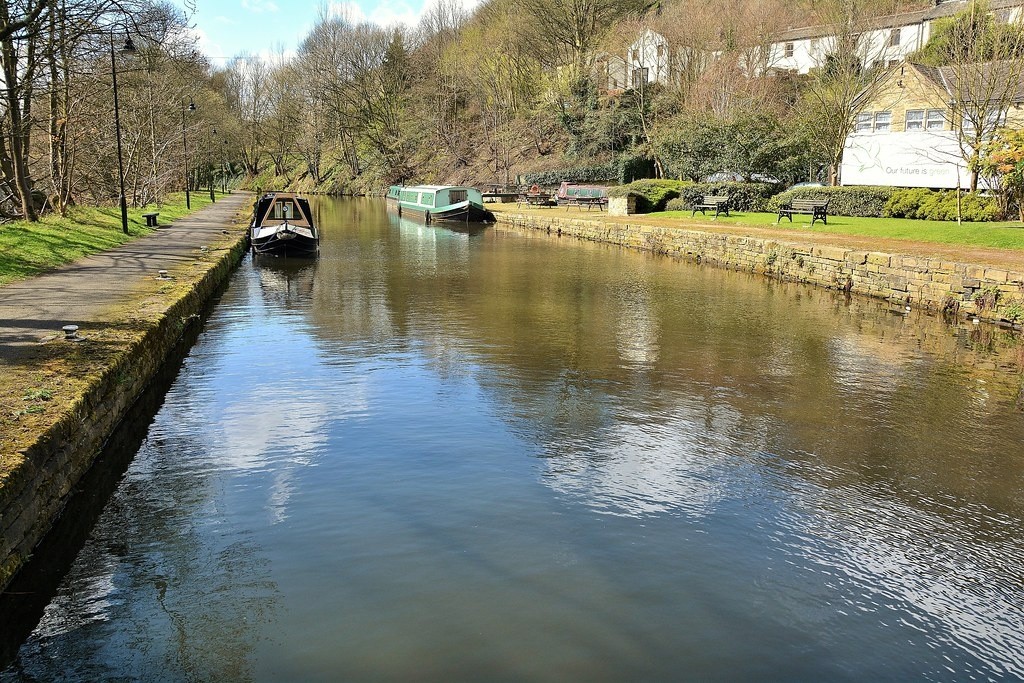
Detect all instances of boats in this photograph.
[250,191,319,255]
[395,185,496,225]
[386,185,400,201]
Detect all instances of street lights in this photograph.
[182,94,197,208]
[111,22,140,231]
[220,135,229,194]
[207,124,218,199]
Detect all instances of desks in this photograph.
[517,196,551,209]
[565,196,606,212]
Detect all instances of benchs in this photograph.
[777,198,830,226]
[563,202,605,205]
[691,196,730,218]
[517,201,554,204]
[143,213,160,227]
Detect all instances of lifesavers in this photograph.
[424,210,429,222]
[531,185,539,193]
[398,205,401,213]
